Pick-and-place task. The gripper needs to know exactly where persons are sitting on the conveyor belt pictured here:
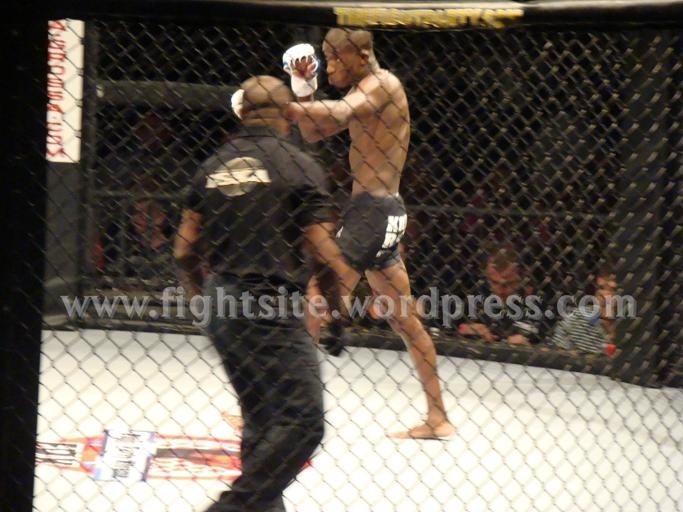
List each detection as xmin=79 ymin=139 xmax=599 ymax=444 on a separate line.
xmin=171 ymin=73 xmax=350 ymax=512
xmin=230 ymin=25 xmax=456 ymax=438
xmin=86 ymin=104 xmax=213 ymax=297
